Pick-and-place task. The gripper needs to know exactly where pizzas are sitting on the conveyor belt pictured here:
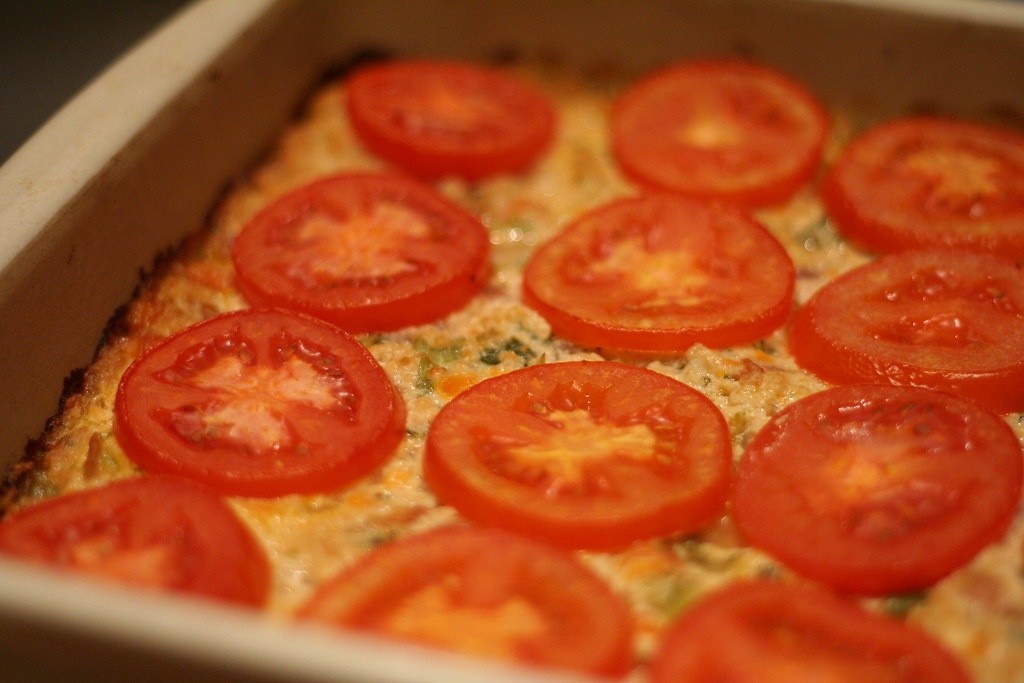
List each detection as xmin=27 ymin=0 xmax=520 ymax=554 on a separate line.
xmin=0 ymin=58 xmax=1024 ymax=683
